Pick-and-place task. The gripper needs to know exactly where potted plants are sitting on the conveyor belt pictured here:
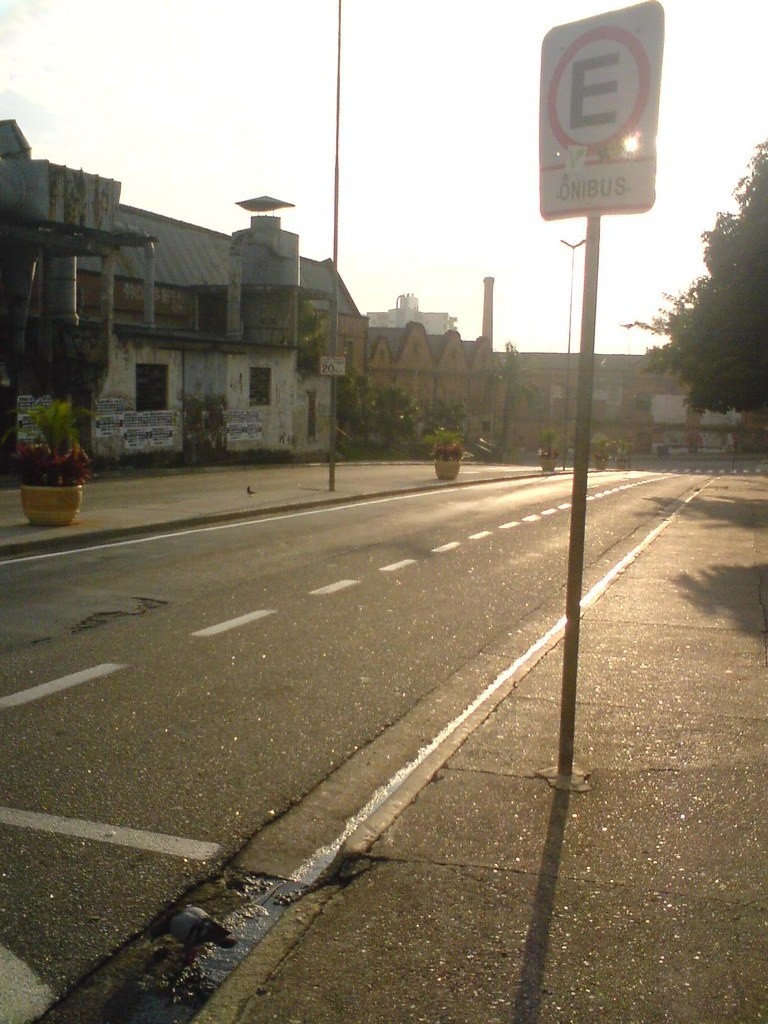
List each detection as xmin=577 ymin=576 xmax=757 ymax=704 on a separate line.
xmin=176 ymin=392 xmax=227 ymax=465
xmin=590 ymin=434 xmax=610 ymax=469
xmin=0 ymin=399 xmax=99 ymax=527
xmin=423 ymin=427 xmax=464 ymax=480
xmin=536 ymin=429 xmax=560 ymax=471
